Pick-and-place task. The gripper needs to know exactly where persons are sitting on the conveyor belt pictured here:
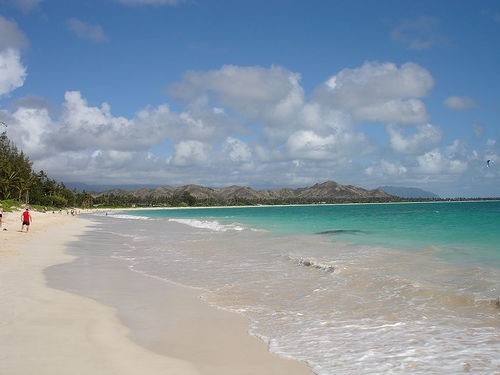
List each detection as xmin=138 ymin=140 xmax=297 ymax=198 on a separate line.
xmin=20 ymin=208 xmax=33 ymax=232
xmin=0 ymin=205 xmax=3 ymax=228
